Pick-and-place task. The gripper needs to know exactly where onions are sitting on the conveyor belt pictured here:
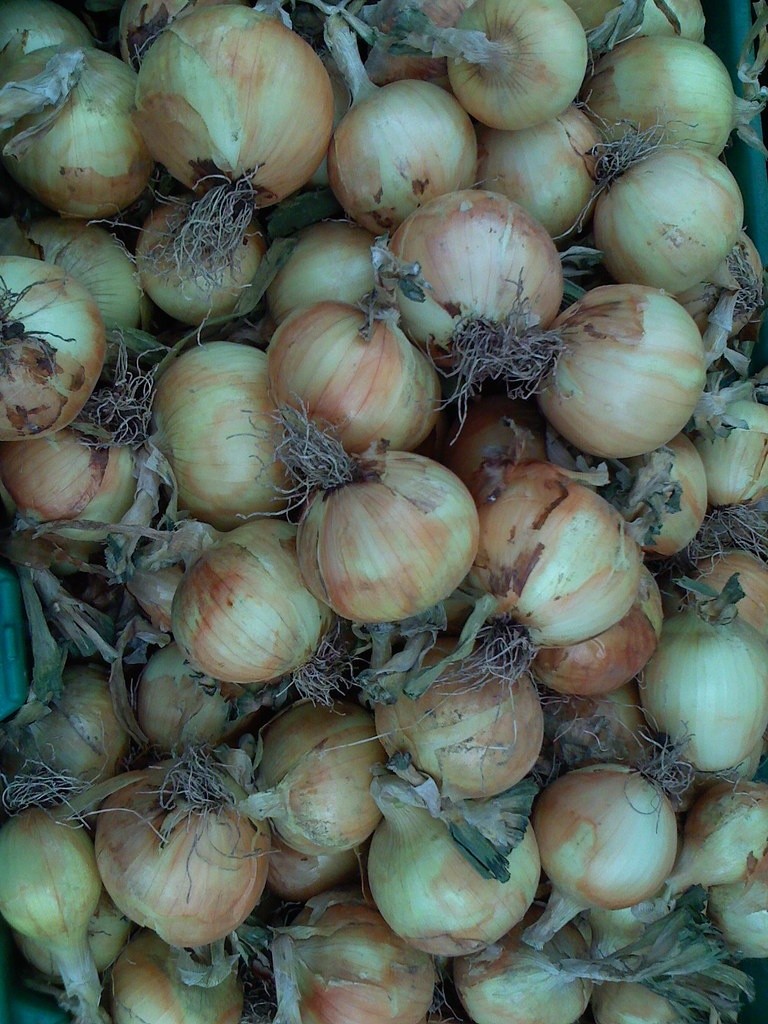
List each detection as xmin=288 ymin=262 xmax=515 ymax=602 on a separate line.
xmin=0 ymin=0 xmax=768 ymax=1024
